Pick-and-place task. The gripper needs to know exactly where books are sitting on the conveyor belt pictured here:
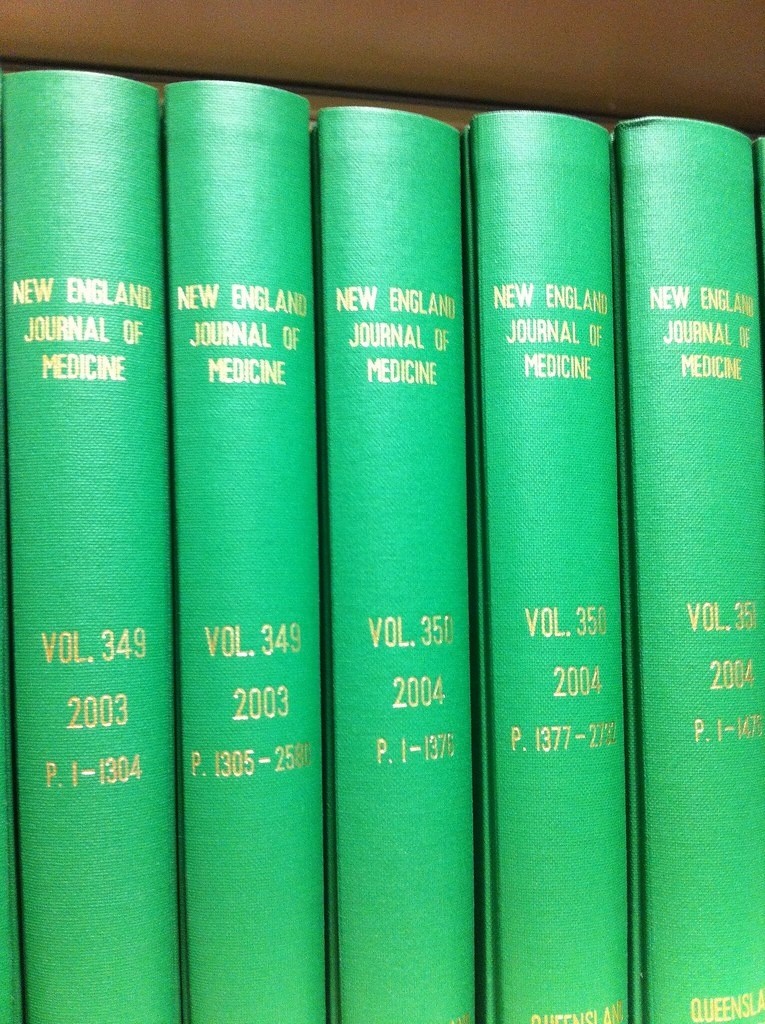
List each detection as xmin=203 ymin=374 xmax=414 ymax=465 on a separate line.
xmin=0 ymin=66 xmax=764 ymax=1024
